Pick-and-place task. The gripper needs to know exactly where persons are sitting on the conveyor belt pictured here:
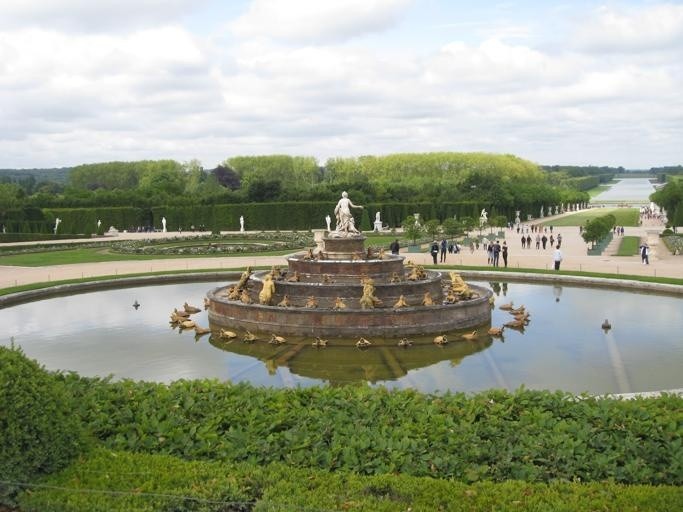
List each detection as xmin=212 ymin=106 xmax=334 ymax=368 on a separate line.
xmin=639 ymin=243 xmax=649 ymax=264
xmin=333 ymin=190 xmax=363 ymax=233
xmin=616 ymin=226 xmax=620 ymax=236
xmin=389 ymin=239 xmax=400 ymax=254
xmin=620 ymin=226 xmax=624 ymax=236
xmin=430 ymin=220 xmax=563 ymax=270
xmin=579 ymin=225 xmax=582 ymax=234
xmin=613 ymin=225 xmax=615 ymax=234
xmin=584 ymin=201 xmax=662 ymax=222
